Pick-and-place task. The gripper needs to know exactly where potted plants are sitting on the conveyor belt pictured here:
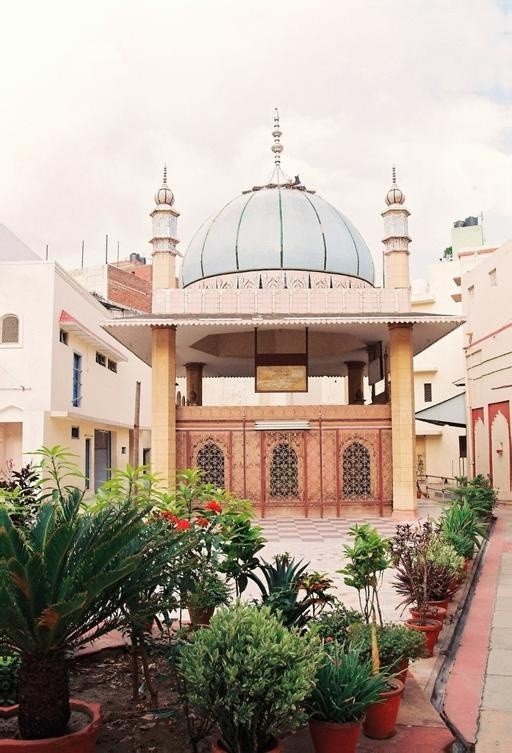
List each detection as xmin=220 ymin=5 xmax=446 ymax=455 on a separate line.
xmin=0 ymin=492 xmax=199 ymax=751
xmin=184 ymin=571 xmax=228 ymax=624
xmin=296 ymin=515 xmax=469 ymax=751
xmin=174 ymin=602 xmax=317 ymax=751
